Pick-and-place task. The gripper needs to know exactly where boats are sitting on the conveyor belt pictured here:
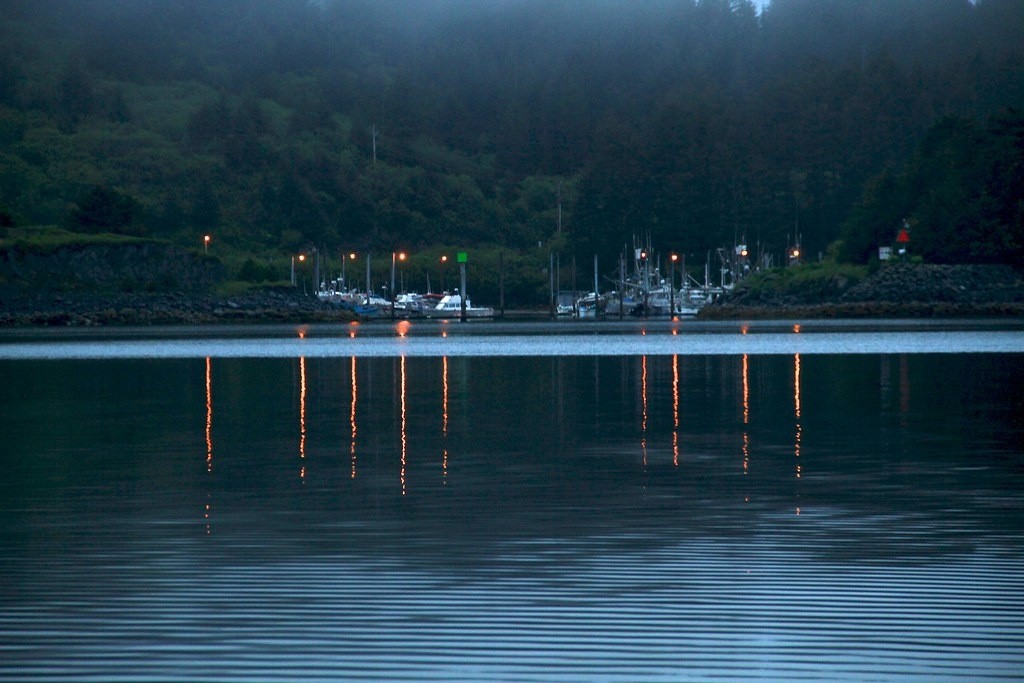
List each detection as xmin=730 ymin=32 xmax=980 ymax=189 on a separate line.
xmin=199 ymin=232 xmax=807 ymax=319
